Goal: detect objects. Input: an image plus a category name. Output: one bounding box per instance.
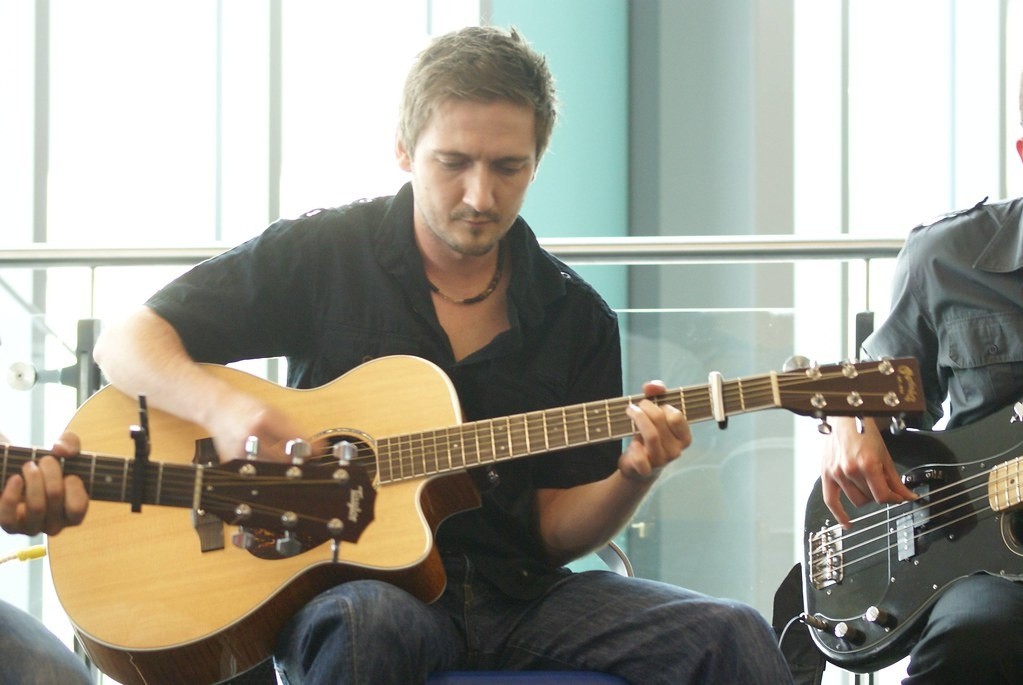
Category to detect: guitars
[802,398,1023,674]
[0,434,378,568]
[43,355,927,685]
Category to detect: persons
[0,430,96,685]
[92,26,793,685]
[821,73,1023,685]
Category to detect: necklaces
[427,239,505,305]
